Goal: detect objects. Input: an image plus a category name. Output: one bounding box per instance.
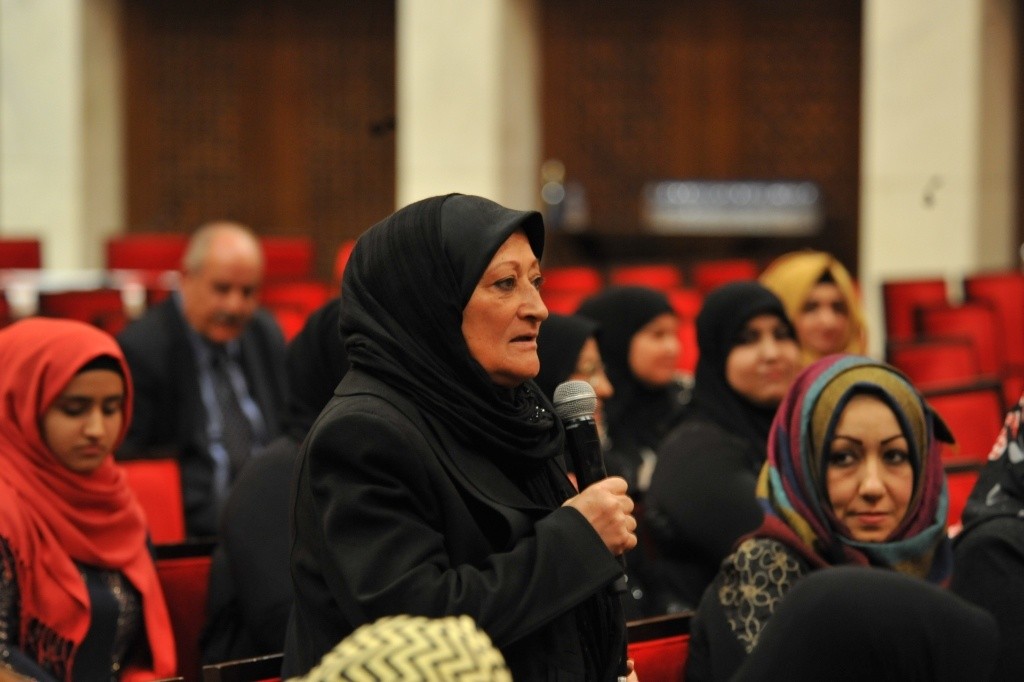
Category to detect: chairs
[1,227,1021,682]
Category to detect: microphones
[554,378,630,594]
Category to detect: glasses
[572,364,607,379]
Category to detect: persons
[682,351,1023,682]
[117,219,288,554]
[530,309,618,492]
[643,277,802,616]
[760,249,866,376]
[280,193,642,682]
[0,316,180,682]
[198,293,352,682]
[571,285,695,624]
